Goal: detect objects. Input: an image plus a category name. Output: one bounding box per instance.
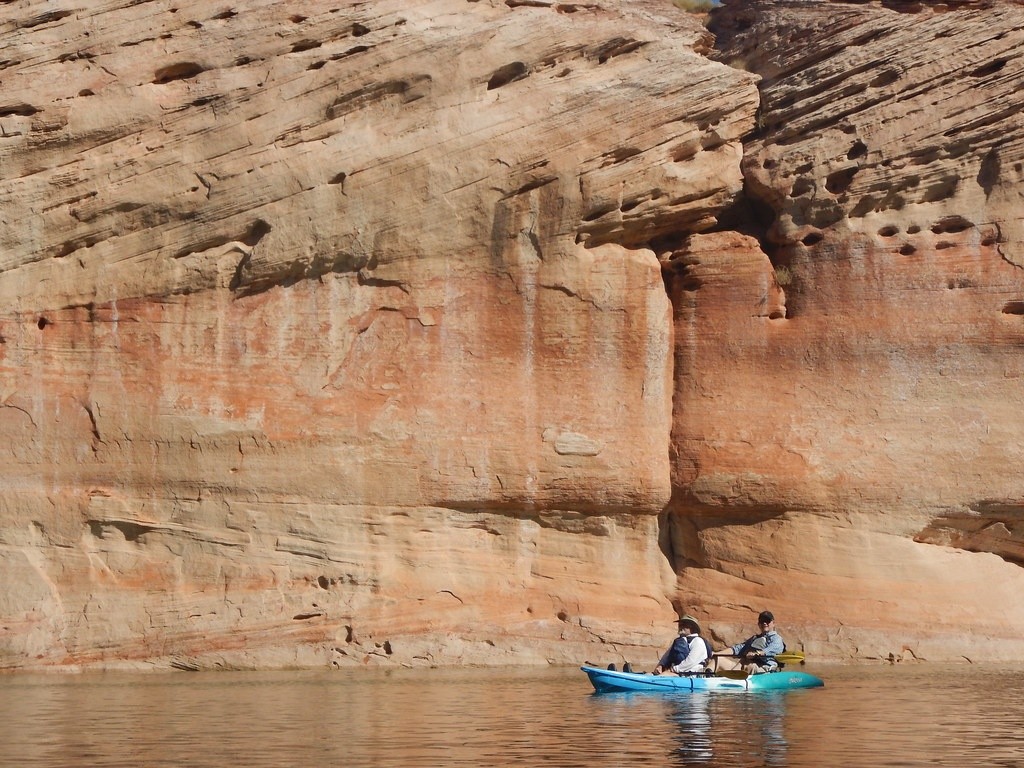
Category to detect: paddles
[714,649,806,664]
[635,669,749,681]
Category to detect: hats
[673,614,700,634]
[758,611,774,622]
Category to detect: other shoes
[705,668,715,678]
[623,663,632,673]
[607,663,617,671]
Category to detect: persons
[606,613,713,678]
[705,610,784,675]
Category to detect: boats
[580,665,825,691]
